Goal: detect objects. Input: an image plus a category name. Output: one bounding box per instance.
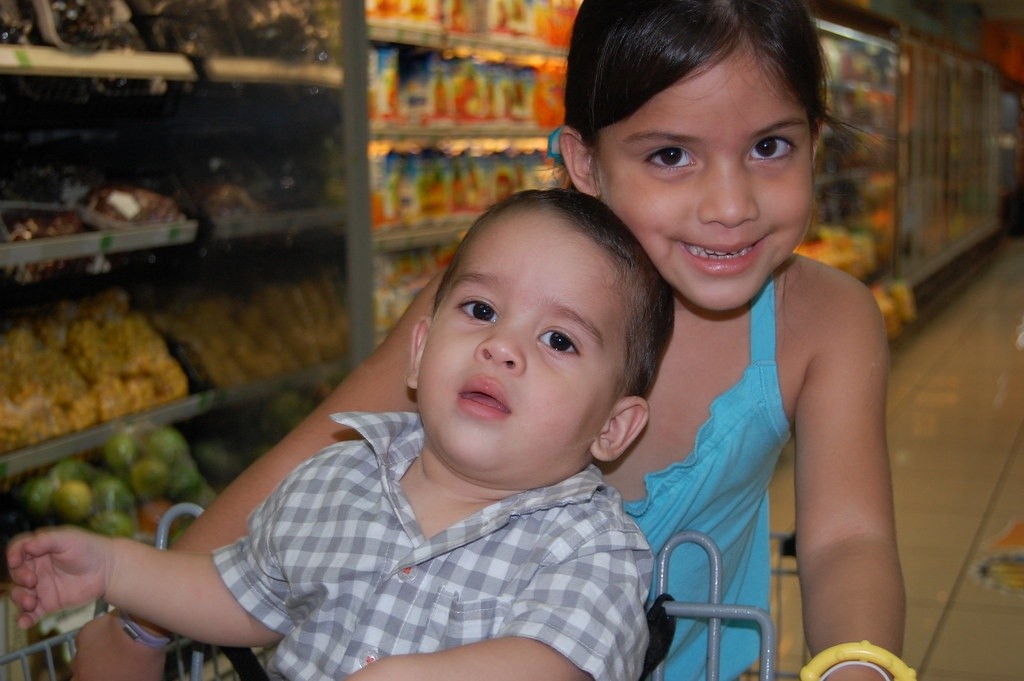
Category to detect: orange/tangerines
[18,381,337,550]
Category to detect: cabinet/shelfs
[0,0,376,681]
[359,0,904,349]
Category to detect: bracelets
[799,640,918,681]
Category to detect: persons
[7,187,676,681]
[69,0,906,681]
